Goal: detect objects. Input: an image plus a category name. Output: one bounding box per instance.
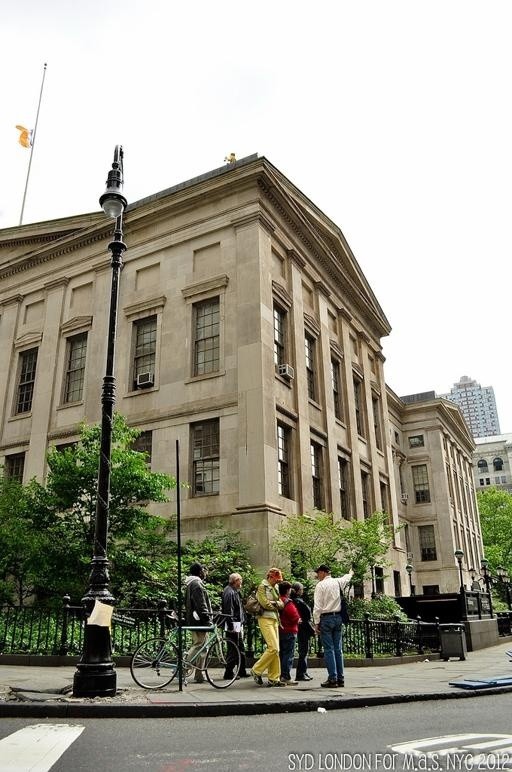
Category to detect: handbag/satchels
[342,596,349,626]
[245,594,264,615]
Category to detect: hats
[314,565,332,573]
[292,582,306,590]
[268,568,283,582]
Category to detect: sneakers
[223,675,240,680]
[237,673,251,678]
[194,678,206,683]
[171,668,184,681]
[251,671,312,686]
[337,678,344,686]
[321,680,338,688]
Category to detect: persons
[291,581,317,681]
[224,153,237,163]
[276,581,302,685]
[172,562,215,686]
[221,573,251,680]
[250,567,287,686]
[311,564,355,688]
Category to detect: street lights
[404,546,495,626]
[67,140,132,698]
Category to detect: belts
[321,612,340,615]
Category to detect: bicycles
[127,609,243,691]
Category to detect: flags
[15,125,34,149]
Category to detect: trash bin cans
[439,623,467,662]
